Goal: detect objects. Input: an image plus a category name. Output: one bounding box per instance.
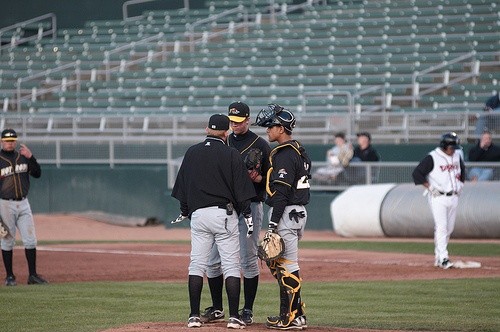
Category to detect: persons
[0,128,48,286]
[200,100,272,326]
[315,131,353,184]
[335,133,379,185]
[256,102,312,330]
[171,114,256,329]
[412,132,466,269]
[468,134,500,162]
[473,90,500,135]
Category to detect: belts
[439,191,457,196]
[218,206,227,209]
[2,196,26,201]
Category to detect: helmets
[440,132,463,150]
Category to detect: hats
[0,128,17,140]
[265,109,296,131]
[228,102,250,122]
[208,113,233,131]
[357,132,370,140]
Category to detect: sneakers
[188,311,204,327]
[203,307,225,323]
[239,309,254,325]
[435,258,454,270]
[272,316,308,330]
[27,274,48,284]
[6,274,16,286]
[227,315,246,328]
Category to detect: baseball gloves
[257,232,285,261]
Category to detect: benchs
[0,0,500,128]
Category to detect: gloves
[244,217,254,238]
[429,186,438,198]
[264,221,278,242]
[171,212,189,224]
